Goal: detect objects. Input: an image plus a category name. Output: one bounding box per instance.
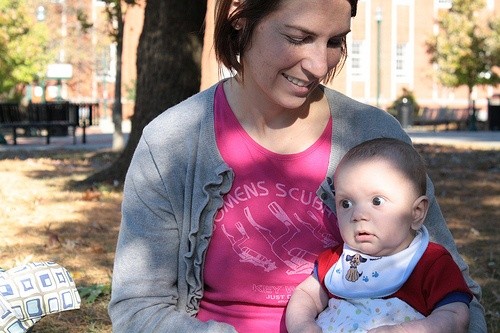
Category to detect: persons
[284,137,473,333]
[107,0,487,333]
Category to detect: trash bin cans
[398,97,413,128]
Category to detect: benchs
[415,107,480,133]
[0,101,99,146]
